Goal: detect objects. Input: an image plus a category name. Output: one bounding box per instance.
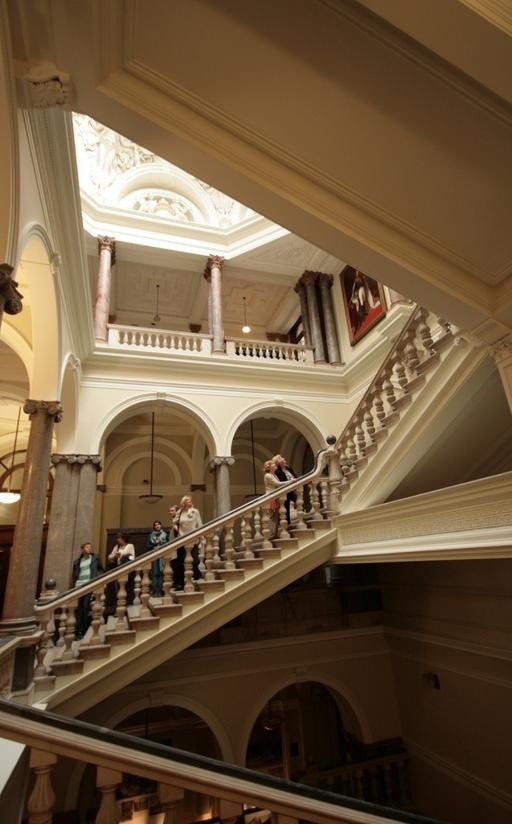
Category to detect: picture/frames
[338,263,390,346]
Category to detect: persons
[262,460,296,540]
[351,271,371,322]
[107,531,136,618]
[72,543,104,641]
[272,454,298,530]
[145,521,169,595]
[169,505,180,541]
[172,495,203,591]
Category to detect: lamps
[135,407,263,508]
[0,402,22,508]
